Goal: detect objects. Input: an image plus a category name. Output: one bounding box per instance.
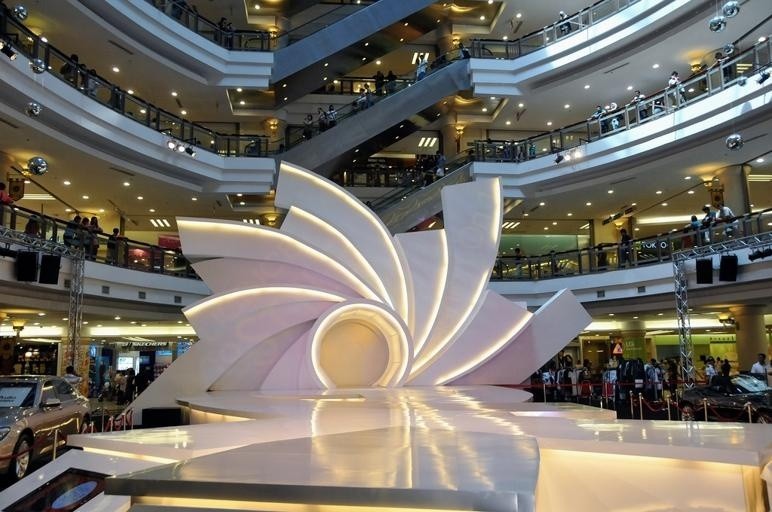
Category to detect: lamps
[167,140,196,157]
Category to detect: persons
[759,459,772,512]
[23,215,40,237]
[112,368,149,402]
[63,216,102,262]
[643,357,695,403]
[619,229,633,268]
[62,366,83,389]
[682,202,738,248]
[539,358,595,405]
[591,103,622,133]
[494,252,502,279]
[2,182,13,204]
[751,354,769,374]
[108,228,120,265]
[514,248,524,277]
[597,246,607,271]
[153,2,234,50]
[707,53,734,83]
[670,71,687,107]
[558,10,572,35]
[630,90,649,120]
[58,53,98,97]
[278,41,493,211]
[703,357,731,386]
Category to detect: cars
[1,374,93,480]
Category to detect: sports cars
[680,375,772,423]
